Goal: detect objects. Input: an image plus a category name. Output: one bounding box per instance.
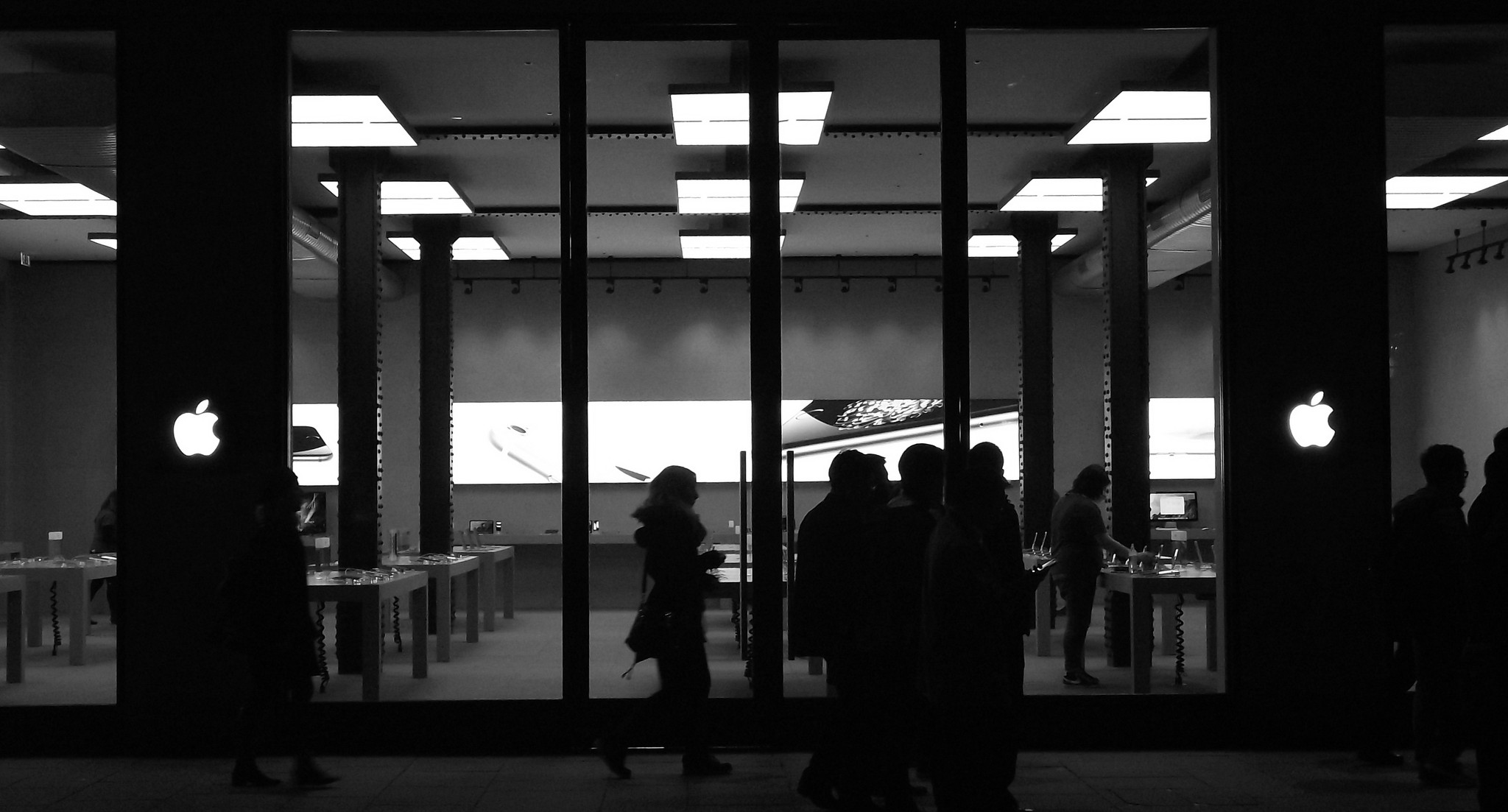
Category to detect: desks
[1099,562,1218,694]
[705,541,753,653]
[304,563,432,700]
[450,544,517,631]
[0,551,119,686]
[377,553,481,663]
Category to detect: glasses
[1461,470,1469,478]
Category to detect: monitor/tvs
[1149,491,1198,521]
[298,492,327,534]
[469,520,494,534]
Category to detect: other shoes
[289,756,342,790]
[1418,759,1475,791]
[231,754,281,787]
[796,749,1018,812]
[90,619,98,625]
[1356,745,1405,766]
[1062,670,1100,689]
[681,751,733,777]
[595,732,633,778]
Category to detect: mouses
[1201,528,1209,530]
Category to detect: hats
[262,467,298,496]
[897,442,945,481]
[969,442,1005,477]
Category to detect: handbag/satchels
[625,600,682,662]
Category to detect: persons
[1051,463,1154,689]
[595,465,733,776]
[1464,427,1508,810]
[229,467,339,791]
[790,442,1051,812]
[1392,444,1473,788]
[87,489,116,626]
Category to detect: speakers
[593,520,600,530]
[496,521,502,531]
